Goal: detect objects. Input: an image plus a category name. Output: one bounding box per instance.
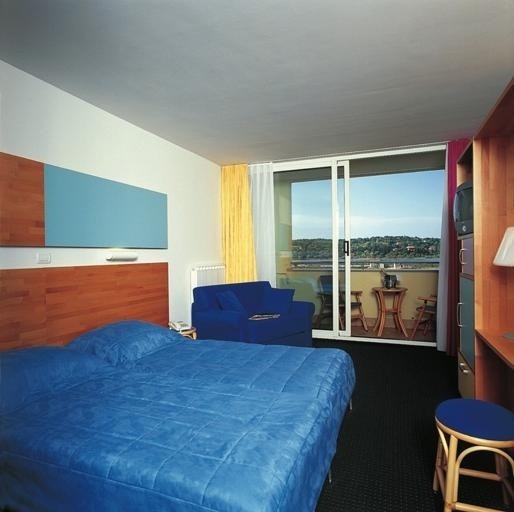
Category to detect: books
[249,314,280,321]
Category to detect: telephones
[167,320,190,331]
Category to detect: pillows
[0,316,183,416]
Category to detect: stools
[316,275,438,340]
[433,398,514,512]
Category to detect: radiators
[192,264,226,288]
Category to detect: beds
[1,261,357,512]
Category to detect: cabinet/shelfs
[456,75,514,409]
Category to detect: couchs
[192,280,315,348]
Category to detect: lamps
[107,252,138,262]
[492,227,514,268]
